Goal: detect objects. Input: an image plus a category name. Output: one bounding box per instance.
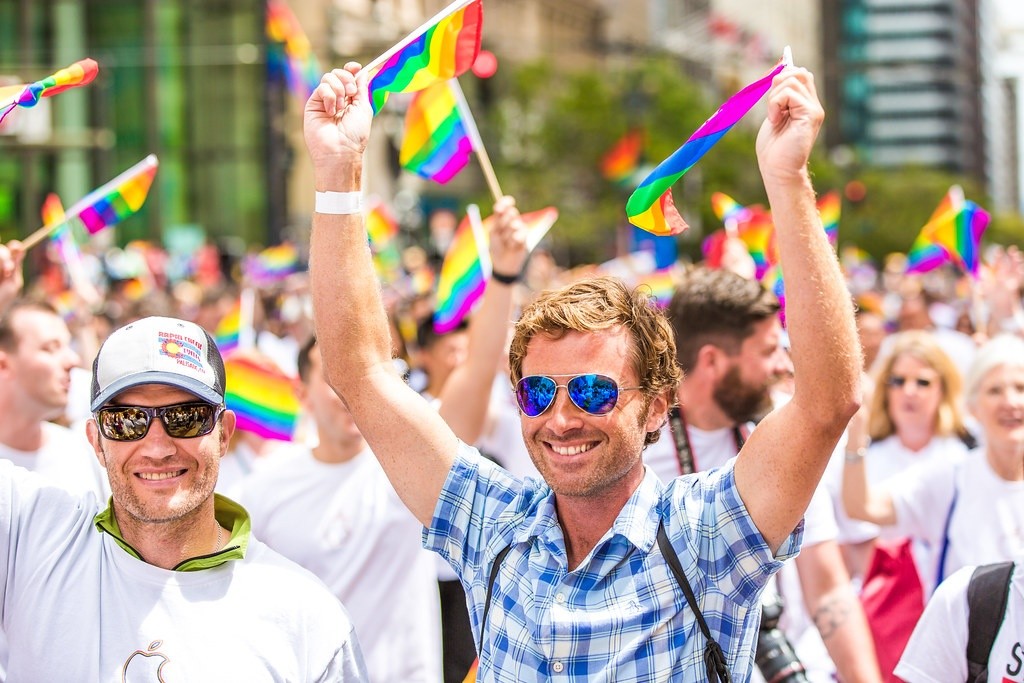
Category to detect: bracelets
[490,271,520,286]
[844,450,864,464]
[311,191,364,215]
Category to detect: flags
[218,348,301,442]
[904,185,991,279]
[361,0,484,185]
[624,62,787,239]
[43,153,157,240]
[0,57,98,114]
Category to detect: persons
[102,410,146,439]
[163,406,213,427]
[0,200,1024,683]
[301,60,865,683]
[523,380,555,410]
[0,238,367,683]
[571,385,618,408]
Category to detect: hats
[90,316,226,412]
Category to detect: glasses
[94,403,226,442]
[512,373,646,417]
